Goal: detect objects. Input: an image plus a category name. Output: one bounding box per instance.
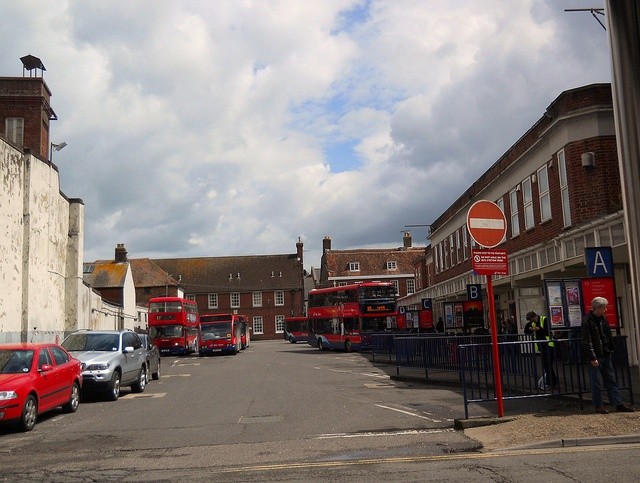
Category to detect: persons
[524,311,556,390]
[581,296,636,413]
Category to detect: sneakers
[595,405,608,414]
[616,403,635,412]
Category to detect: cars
[137,334,161,384]
[0,343,83,431]
[59,328,147,401]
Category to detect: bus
[198,314,241,357]
[308,281,398,353]
[237,315,250,350]
[284,317,308,343]
[147,297,199,356]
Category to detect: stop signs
[467,200,508,249]
